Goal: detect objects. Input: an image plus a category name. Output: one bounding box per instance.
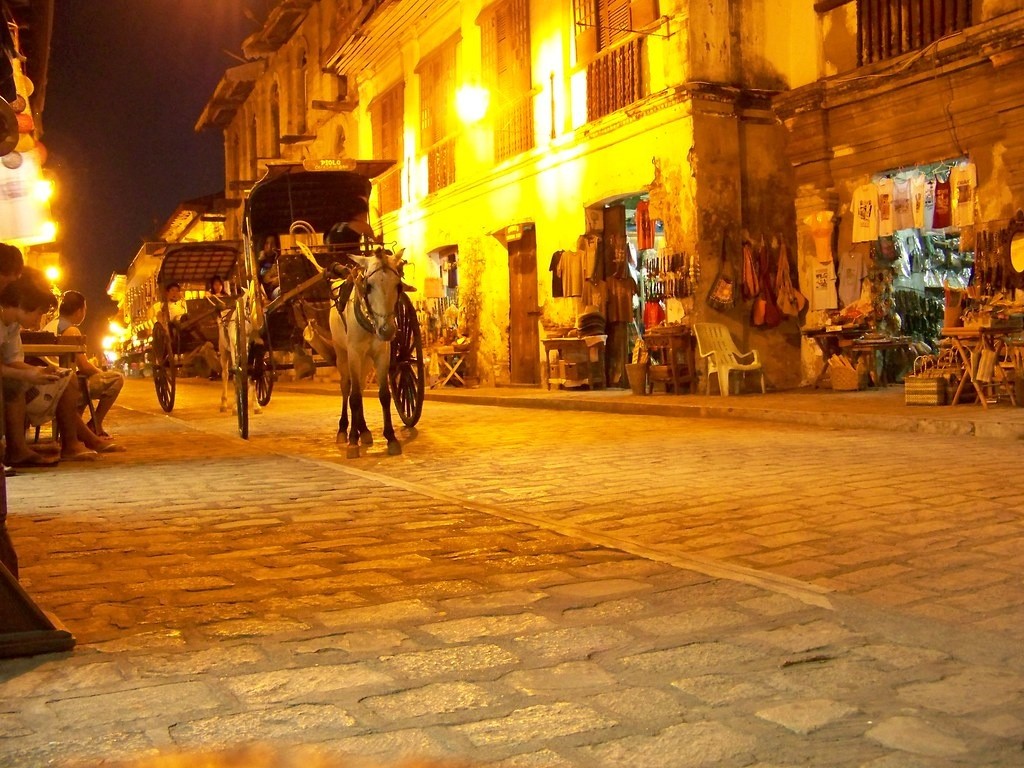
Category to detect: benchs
[186,299,220,342]
[280,251,340,301]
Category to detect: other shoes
[4,466,16,476]
[4,452,59,467]
[98,431,115,440]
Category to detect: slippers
[58,450,104,462]
[98,444,126,453]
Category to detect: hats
[776,285,807,313]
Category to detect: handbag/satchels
[706,274,736,312]
[743,243,760,296]
[752,297,767,326]
[764,303,784,328]
[740,279,751,302]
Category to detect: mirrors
[998,212,1024,289]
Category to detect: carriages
[104,237,278,418]
[228,156,429,459]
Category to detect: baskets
[904,344,972,405]
[827,362,867,392]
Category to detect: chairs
[35,374,105,443]
[694,322,765,398]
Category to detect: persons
[0,241,145,475]
[167,285,213,348]
[203,275,230,297]
[803,211,838,269]
[257,233,282,275]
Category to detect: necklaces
[2,304,12,343]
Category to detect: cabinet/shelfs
[541,335,607,392]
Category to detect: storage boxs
[649,364,671,379]
[963,316,1023,328]
[558,360,578,380]
[550,364,559,378]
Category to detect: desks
[806,332,875,384]
[940,328,1020,406]
[438,350,472,387]
[643,333,697,396]
[25,343,88,443]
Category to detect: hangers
[863,173,869,184]
[932,159,954,174]
[914,162,921,172]
[881,167,888,179]
[898,164,904,173]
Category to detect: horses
[329,248,416,459]
[217,279,262,415]
